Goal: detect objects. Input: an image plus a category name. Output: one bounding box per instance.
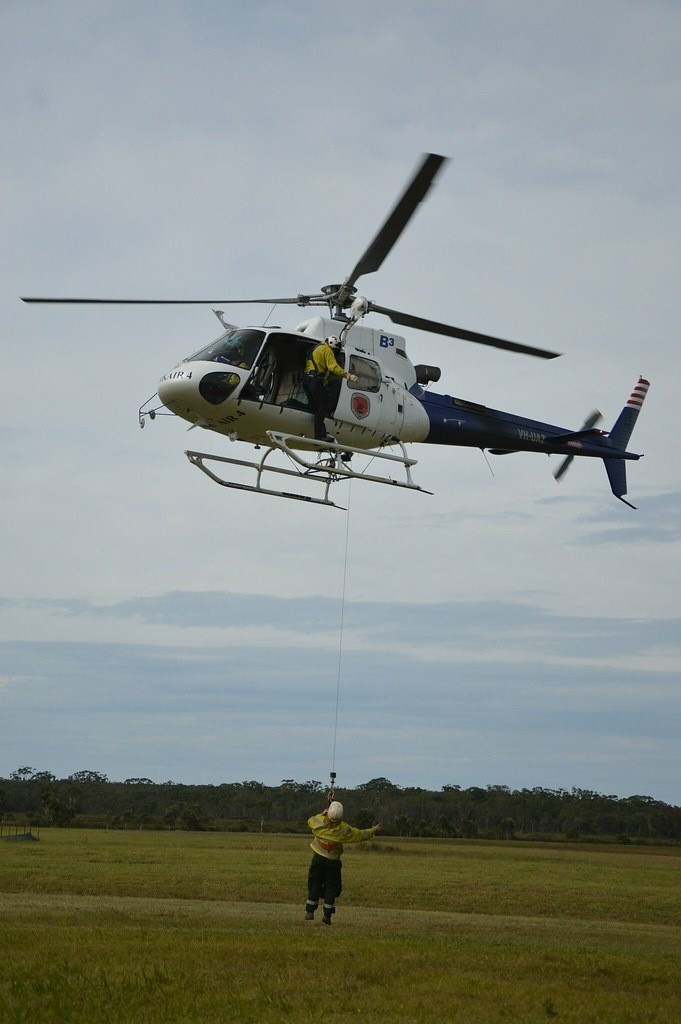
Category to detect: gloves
[347,373,359,384]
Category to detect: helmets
[328,335,338,348]
[328,801,343,822]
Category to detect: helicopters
[22,153,651,512]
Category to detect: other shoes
[313,436,334,446]
[322,916,331,925]
[305,913,314,920]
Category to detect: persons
[218,352,251,396]
[304,802,382,924]
[303,335,359,445]
